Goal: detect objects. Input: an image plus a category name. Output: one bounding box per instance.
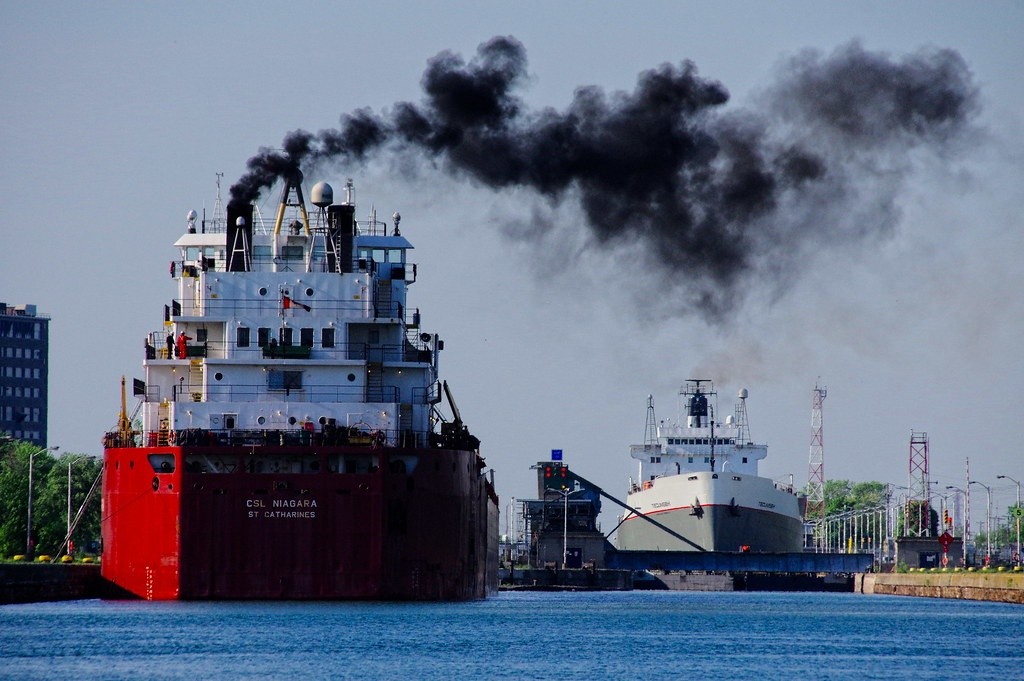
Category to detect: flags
[283,296,312,312]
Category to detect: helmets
[171,332,174,335]
[181,331,185,334]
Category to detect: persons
[166,331,192,360]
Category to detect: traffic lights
[67,540,74,555]
[545,466,569,482]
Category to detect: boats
[101,159,501,601]
[618,379,803,553]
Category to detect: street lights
[66,455,97,554]
[547,487,586,563]
[805,475,1020,568]
[26,446,60,561]
